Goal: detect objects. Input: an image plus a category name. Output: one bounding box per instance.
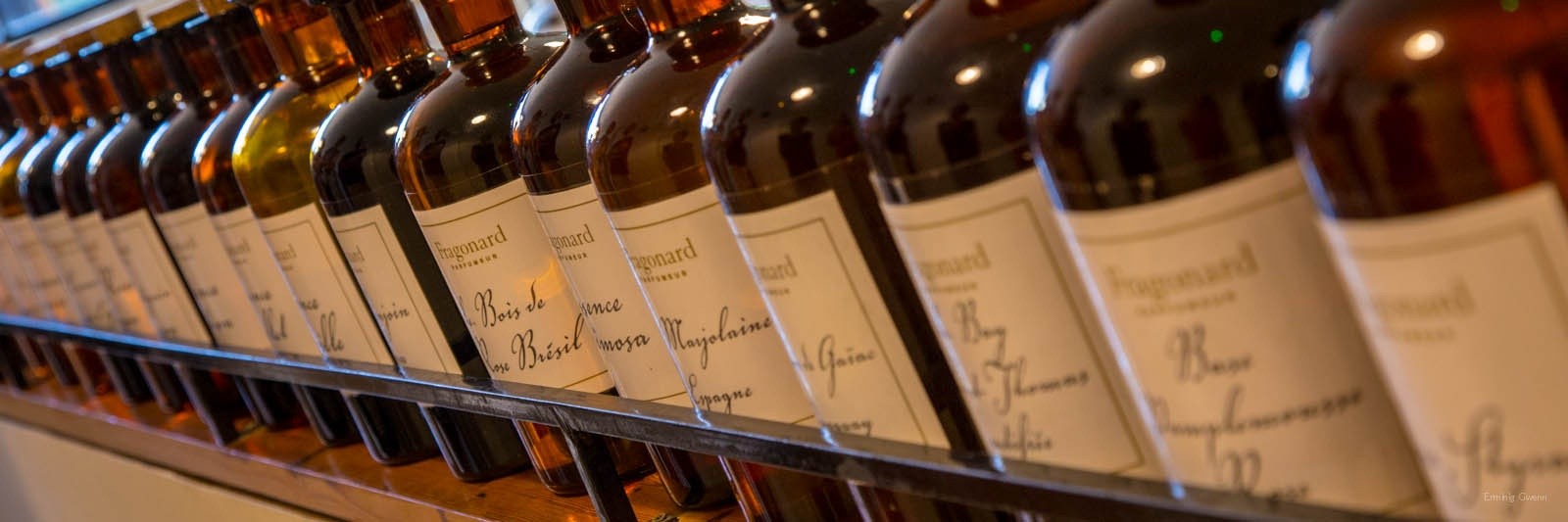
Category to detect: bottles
[1020,0,1440,522]
[699,0,1010,522]
[0,0,189,418]
[508,0,738,512]
[135,0,306,434]
[854,0,1166,522]
[308,0,530,485]
[230,0,443,466]
[189,0,361,449]
[1279,0,1568,522]
[392,0,658,500]
[584,0,853,522]
